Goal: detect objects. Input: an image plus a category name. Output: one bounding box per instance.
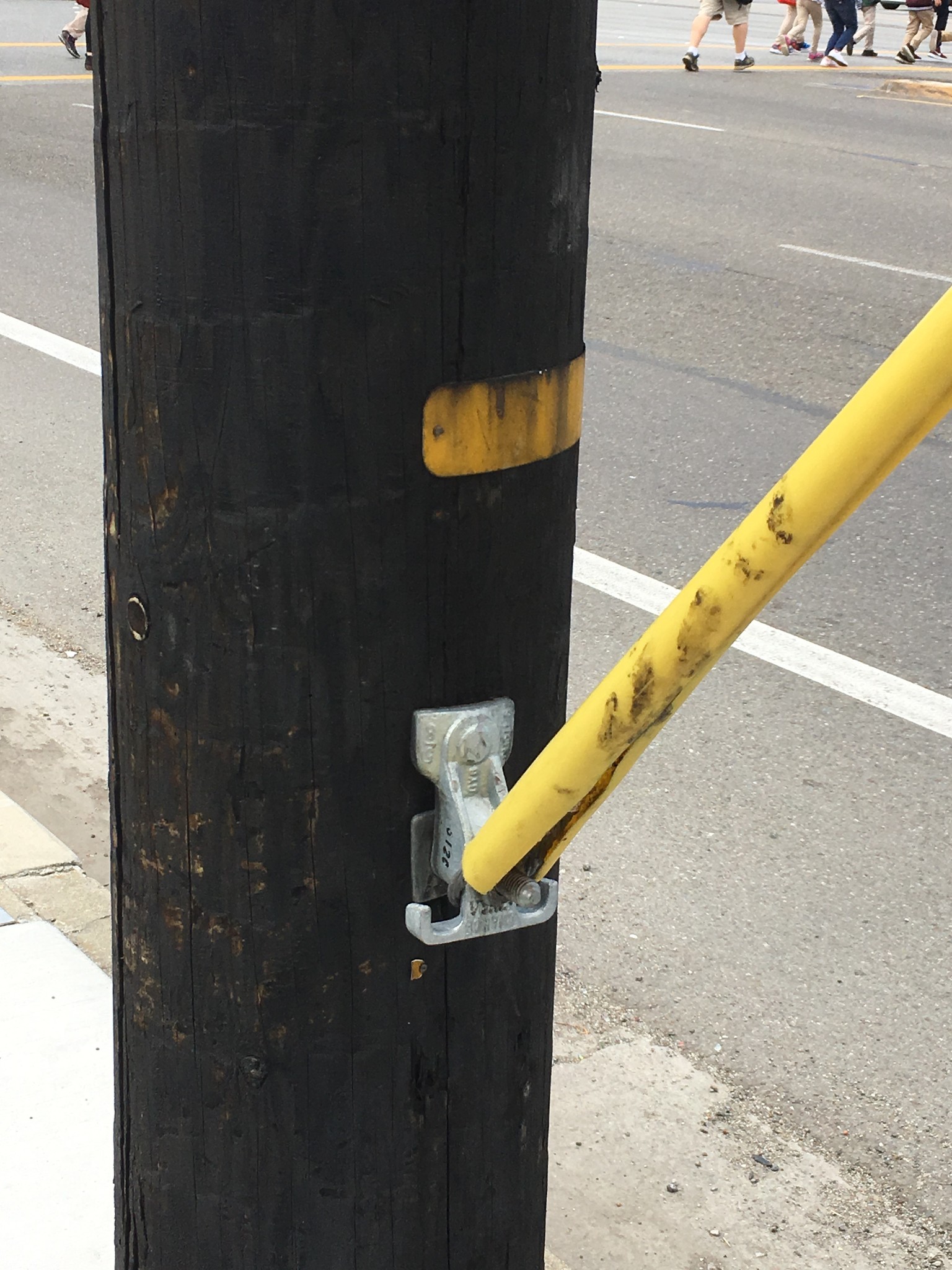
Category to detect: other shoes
[846,37,856,55]
[929,30,942,51]
[862,50,877,56]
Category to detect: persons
[682,0,755,71]
[58,0,93,70]
[769,0,952,69]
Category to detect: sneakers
[807,52,823,61]
[828,49,847,66]
[85,54,93,69]
[771,44,783,54]
[682,53,700,71]
[928,50,947,60]
[820,56,838,67]
[913,54,922,60]
[895,52,912,64]
[59,31,80,58]
[734,56,754,70]
[800,42,810,50]
[903,43,915,62]
[789,40,802,53]
[780,35,790,56]
